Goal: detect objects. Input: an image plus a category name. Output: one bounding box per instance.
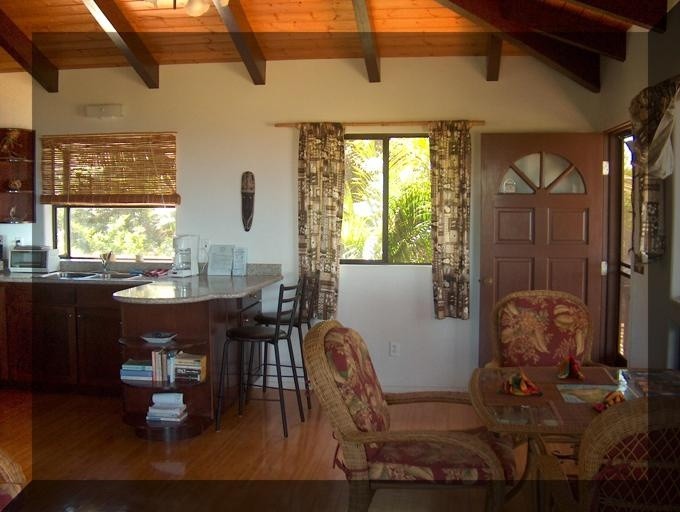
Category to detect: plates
[141,330,178,344]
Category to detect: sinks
[86,273,143,281]
[42,271,97,281]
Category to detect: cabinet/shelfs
[238,289,262,392]
[119,336,208,430]
[6,283,139,395]
[0,128,35,224]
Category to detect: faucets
[100,250,113,274]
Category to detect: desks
[468,366,680,512]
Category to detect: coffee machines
[168,233,201,278]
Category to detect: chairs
[245,269,320,410]
[0,447,32,512]
[531,397,680,512]
[484,290,618,367]
[215,278,305,437]
[302,321,516,512]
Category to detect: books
[620,366,680,403]
[119,348,207,421]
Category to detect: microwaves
[7,248,61,274]
